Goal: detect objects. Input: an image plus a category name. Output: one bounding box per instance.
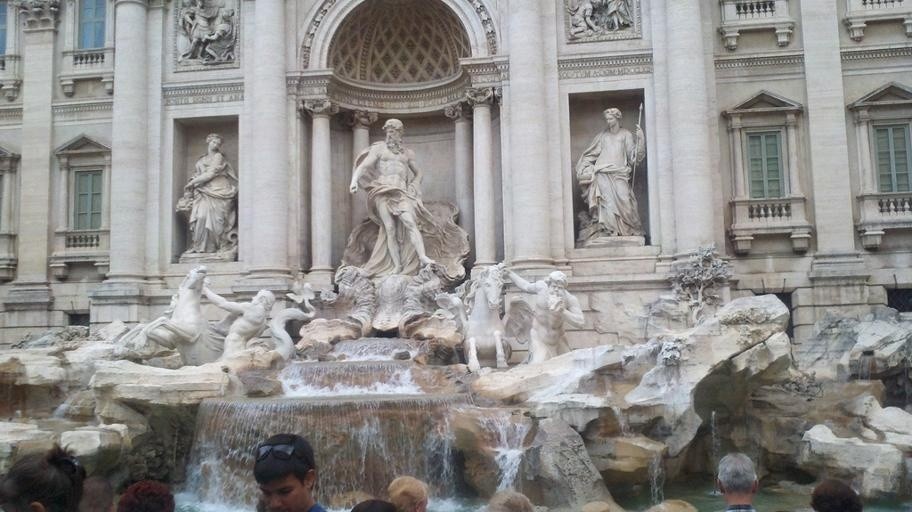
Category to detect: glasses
[257,444,295,460]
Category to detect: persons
[78,477,114,512]
[810,478,863,512]
[351,117,468,275]
[179,0,236,63]
[183,133,238,252]
[388,476,428,512]
[118,482,175,512]
[0,445,86,512]
[575,108,647,238]
[566,0,635,42]
[202,280,315,367]
[490,491,534,512]
[502,266,585,365]
[718,452,760,512]
[350,499,395,512]
[255,433,326,512]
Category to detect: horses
[117,264,225,365]
[460,261,511,374]
